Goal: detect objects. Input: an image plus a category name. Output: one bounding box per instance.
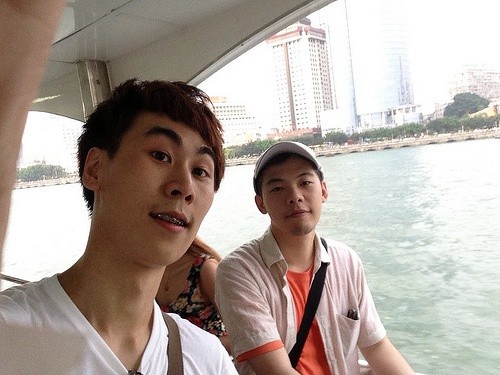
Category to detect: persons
[215,142,415,375]
[0,0,239,375]
[155,235,232,356]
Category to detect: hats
[253,142,325,182]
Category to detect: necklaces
[165,267,171,291]
